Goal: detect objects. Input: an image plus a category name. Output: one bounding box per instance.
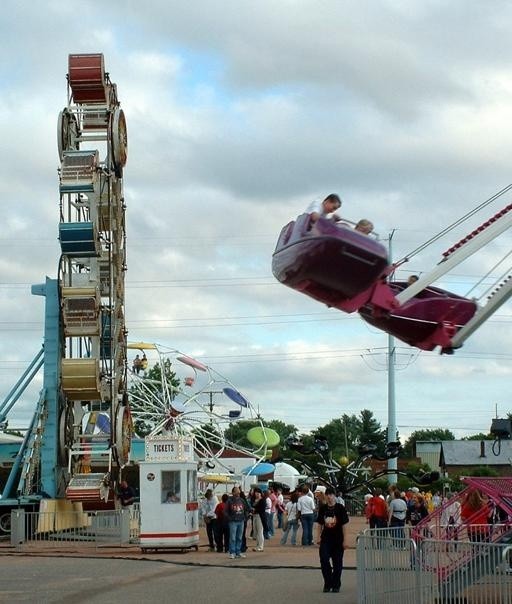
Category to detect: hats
[408,487,419,493]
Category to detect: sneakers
[209,547,263,559]
[332,588,339,592]
[323,587,331,591]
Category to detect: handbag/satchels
[283,516,287,532]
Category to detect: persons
[364,486,462,553]
[356,220,374,235]
[165,492,175,504]
[405,275,419,286]
[304,194,342,222]
[461,488,509,559]
[201,488,266,559]
[132,354,147,375]
[315,488,349,593]
[263,485,345,546]
[116,479,135,521]
